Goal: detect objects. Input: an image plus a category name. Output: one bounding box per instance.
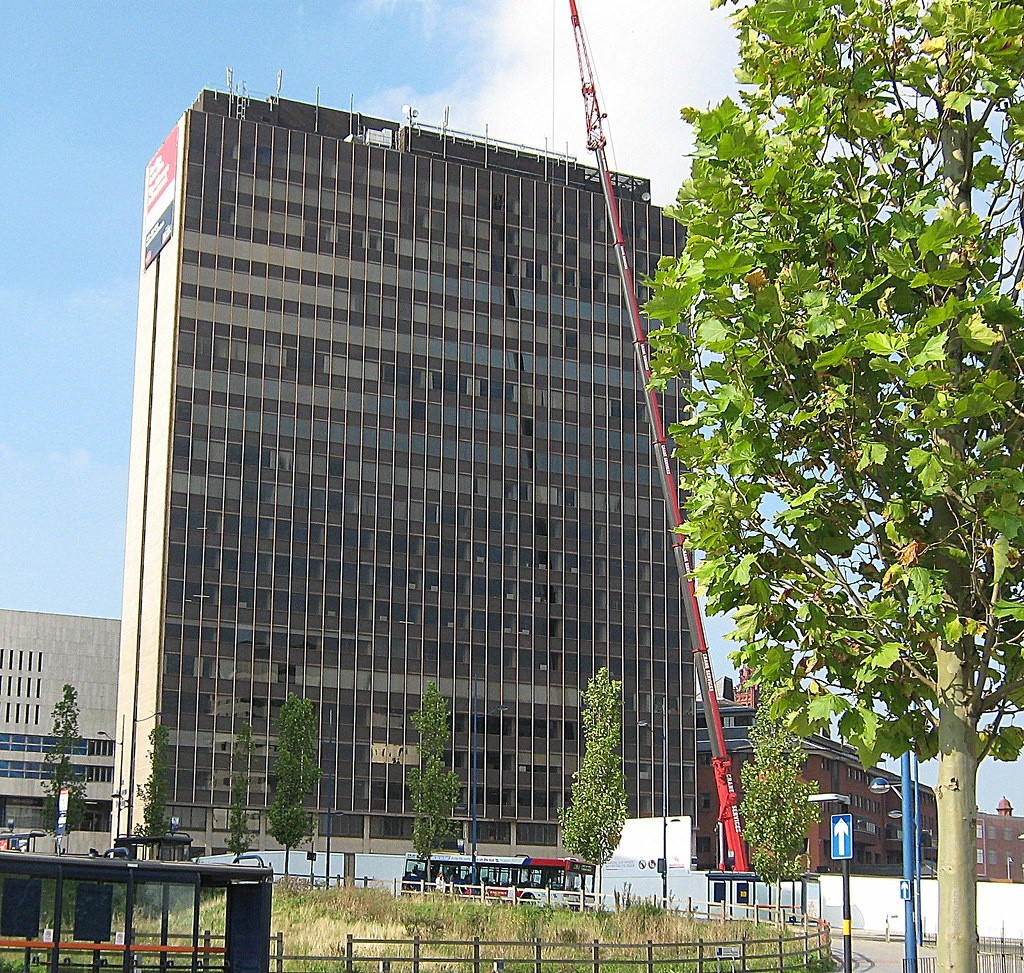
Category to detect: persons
[435,872,446,894]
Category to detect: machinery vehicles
[569,1,750,872]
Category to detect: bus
[400,852,595,913]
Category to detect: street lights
[326,811,344,887]
[638,697,668,908]
[472,673,508,894]
[128,711,169,837]
[869,776,923,948]
[97,714,126,838]
